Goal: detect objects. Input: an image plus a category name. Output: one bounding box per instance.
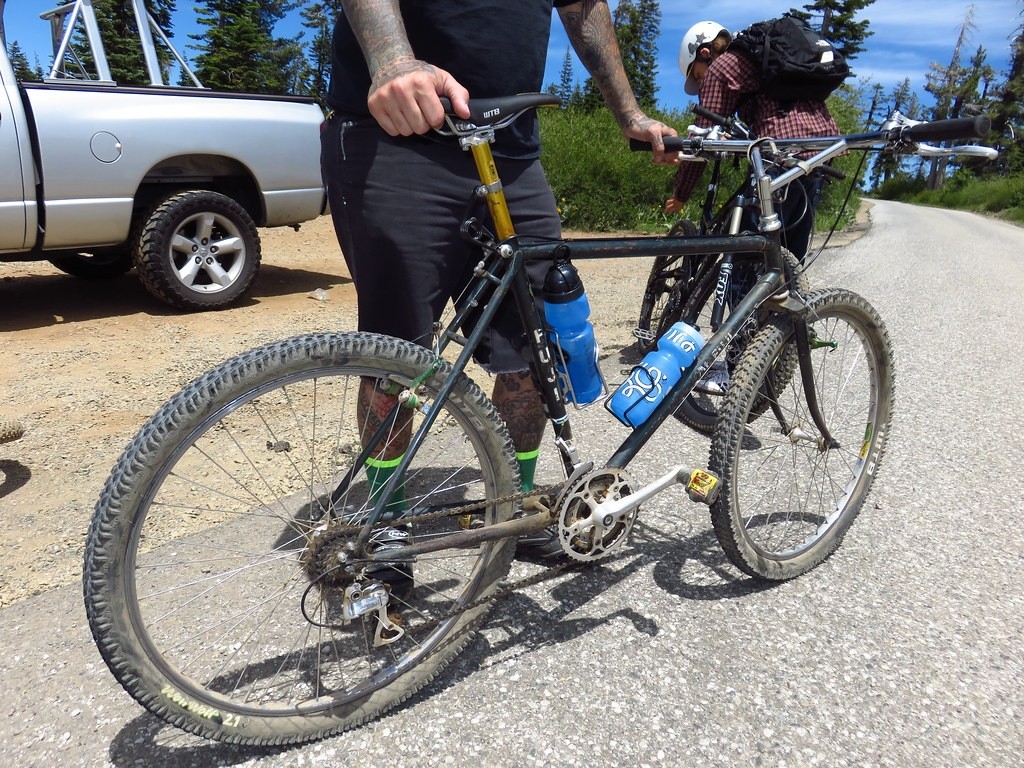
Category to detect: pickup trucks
[0,1,333,310]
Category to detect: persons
[661,16,850,391]
[321,0,681,616]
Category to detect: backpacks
[727,11,850,116]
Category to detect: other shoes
[513,511,577,560]
[370,523,416,603]
[693,367,729,395]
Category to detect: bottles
[541,244,603,405]
[609,321,704,425]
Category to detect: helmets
[678,20,732,95]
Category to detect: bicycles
[82,92,895,748]
[631,103,848,432]
